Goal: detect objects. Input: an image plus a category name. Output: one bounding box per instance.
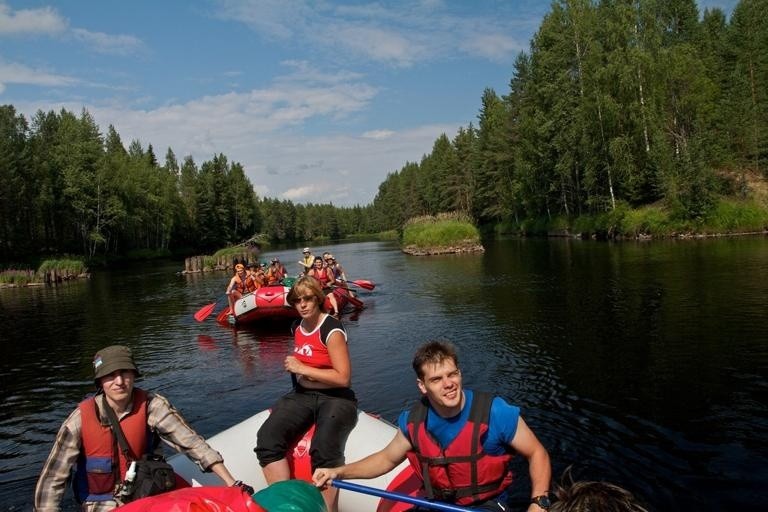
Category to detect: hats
[303,248,311,254]
[324,255,335,260]
[235,256,279,269]
[92,345,136,380]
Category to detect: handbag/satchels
[121,453,176,504]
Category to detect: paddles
[217,280,255,325]
[322,281,364,309]
[194,274,250,322]
[339,279,375,290]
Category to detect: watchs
[531,495,551,508]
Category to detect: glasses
[292,295,316,304]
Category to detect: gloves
[232,481,254,495]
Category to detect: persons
[547,464,645,510]
[309,338,552,512]
[224,247,356,319]
[30,343,254,511]
[254,275,360,511]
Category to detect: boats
[161,402,427,512]
[230,284,349,327]
